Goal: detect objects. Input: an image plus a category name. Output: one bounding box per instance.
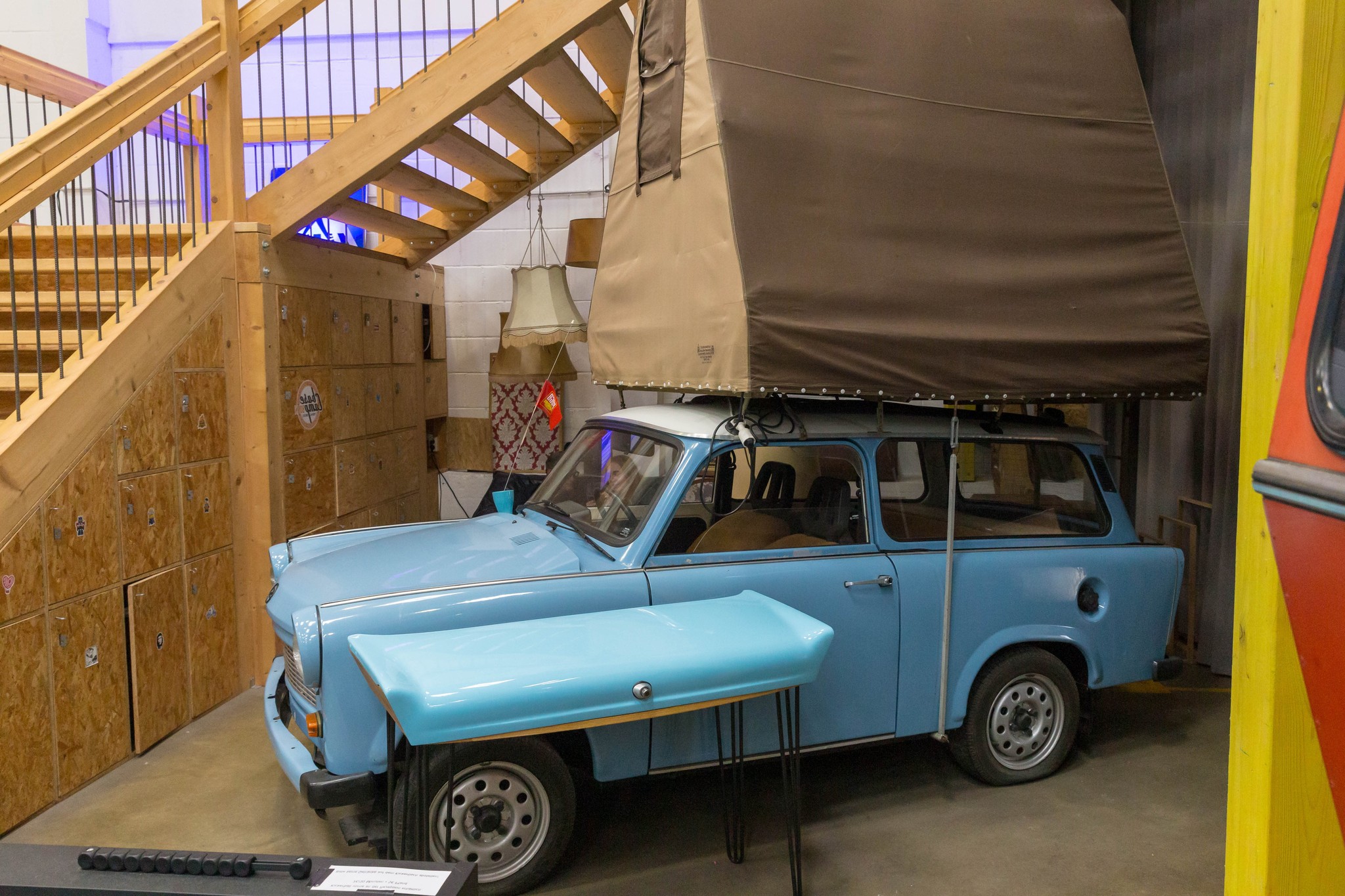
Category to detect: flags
[535,379,563,431]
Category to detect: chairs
[682,461,850,554]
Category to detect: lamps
[487,192,578,382]
[564,101,606,268]
[502,184,587,350]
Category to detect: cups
[492,489,514,514]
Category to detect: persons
[585,454,647,508]
[512,451,583,515]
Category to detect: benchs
[880,499,1076,536]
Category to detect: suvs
[258,396,1184,896]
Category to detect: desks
[347,591,835,896]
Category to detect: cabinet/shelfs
[0,282,492,840]
